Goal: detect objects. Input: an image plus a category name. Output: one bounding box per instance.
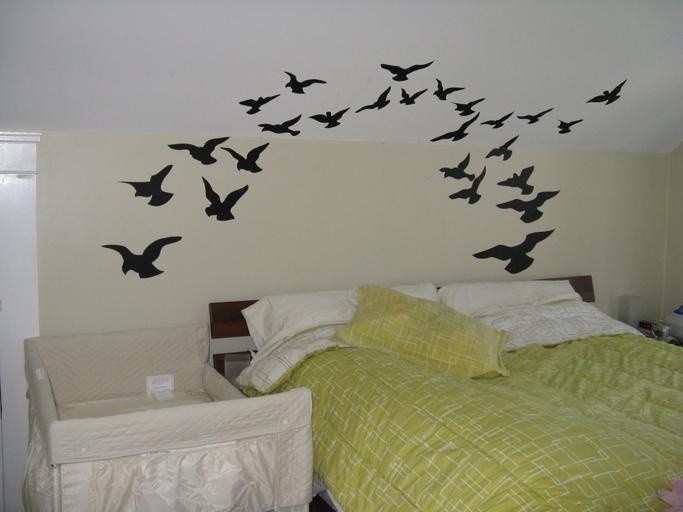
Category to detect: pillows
[336,284,510,378]
[242,283,440,356]
[436,280,585,318]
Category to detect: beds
[208,275,683,512]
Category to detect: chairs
[18,321,313,512]
